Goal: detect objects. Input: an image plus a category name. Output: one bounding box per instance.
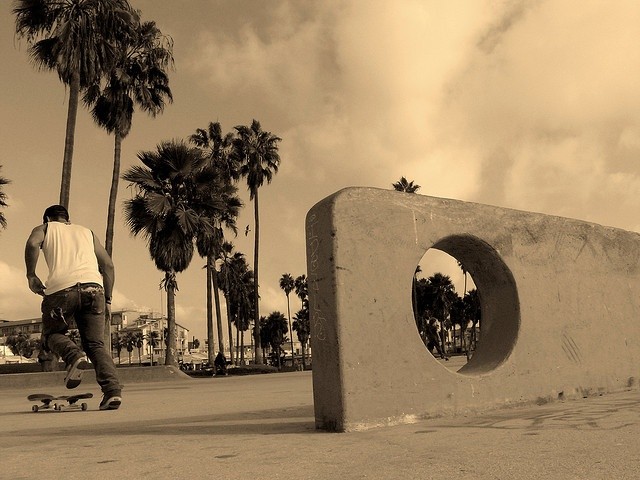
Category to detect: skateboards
[28,393,93,411]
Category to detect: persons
[24,204,122,410]
[212,352,228,375]
[424,317,449,359]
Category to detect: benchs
[201,361,240,376]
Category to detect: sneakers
[64,355,88,389]
[99,389,122,411]
